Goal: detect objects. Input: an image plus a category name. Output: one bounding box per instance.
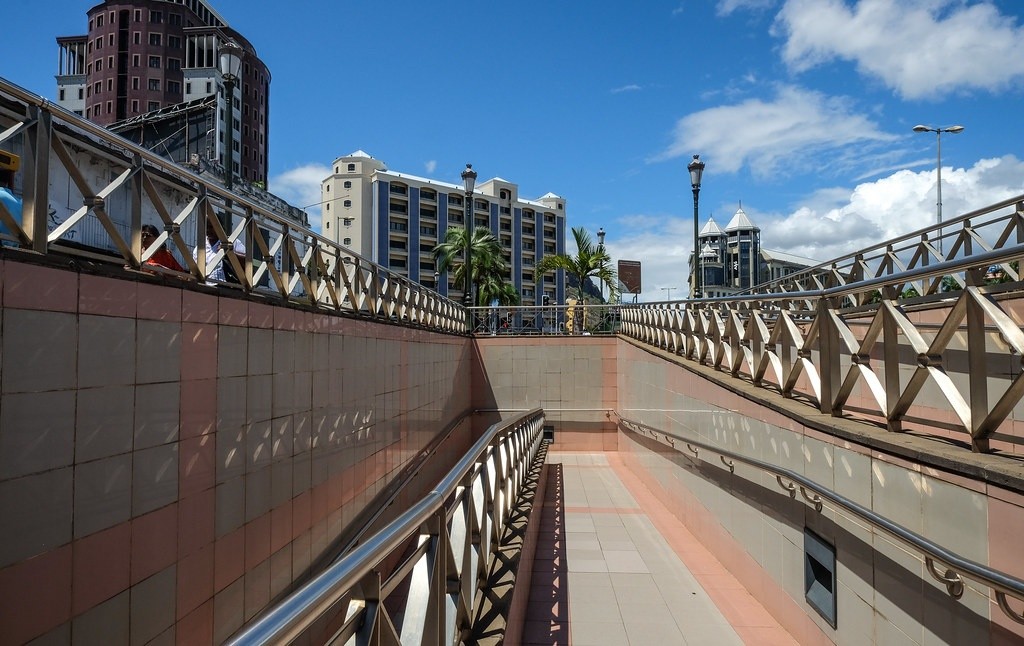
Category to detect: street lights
[687,154,706,298]
[336,216,355,243]
[460,163,478,333]
[218,36,244,235]
[597,227,606,305]
[912,124,964,293]
[435,271,439,293]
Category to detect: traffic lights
[542,295,550,313]
[507,312,512,324]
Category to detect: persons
[141,224,184,275]
[583,329,591,336]
[503,321,508,335]
[191,213,246,288]
